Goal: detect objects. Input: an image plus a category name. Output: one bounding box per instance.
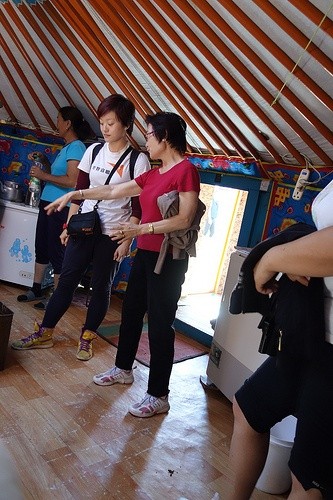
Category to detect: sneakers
[10,321,53,350]
[92,367,134,387]
[75,330,98,360]
[127,393,170,418]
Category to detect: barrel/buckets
[254,415,297,494]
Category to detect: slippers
[33,301,48,309]
[17,291,45,302]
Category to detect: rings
[32,171,34,174]
[122,235,125,238]
[120,231,123,235]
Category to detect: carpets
[81,319,210,370]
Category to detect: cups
[25,188,40,206]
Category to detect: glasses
[143,130,158,140]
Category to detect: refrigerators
[206,246,270,406]
[0,199,40,288]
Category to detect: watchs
[63,223,68,230]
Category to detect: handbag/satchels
[65,211,102,237]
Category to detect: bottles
[28,154,43,194]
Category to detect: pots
[0,180,26,202]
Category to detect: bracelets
[148,222,155,235]
[78,190,84,201]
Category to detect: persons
[16,105,91,311]
[11,92,152,361]
[44,110,201,419]
[211,174,333,500]
